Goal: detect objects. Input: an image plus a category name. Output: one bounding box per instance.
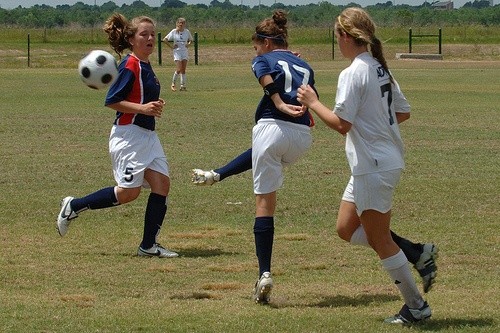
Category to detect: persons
[163,17,193,90]
[190,11,319,305]
[296,8,441,327]
[55,13,180,259]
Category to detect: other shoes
[171,84,177,91]
[180,85,187,91]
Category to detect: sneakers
[252,272,273,304]
[412,243,439,293]
[137,243,179,258]
[188,168,221,188]
[54,195,79,237]
[383,300,432,326]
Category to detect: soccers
[78,50,119,91]
